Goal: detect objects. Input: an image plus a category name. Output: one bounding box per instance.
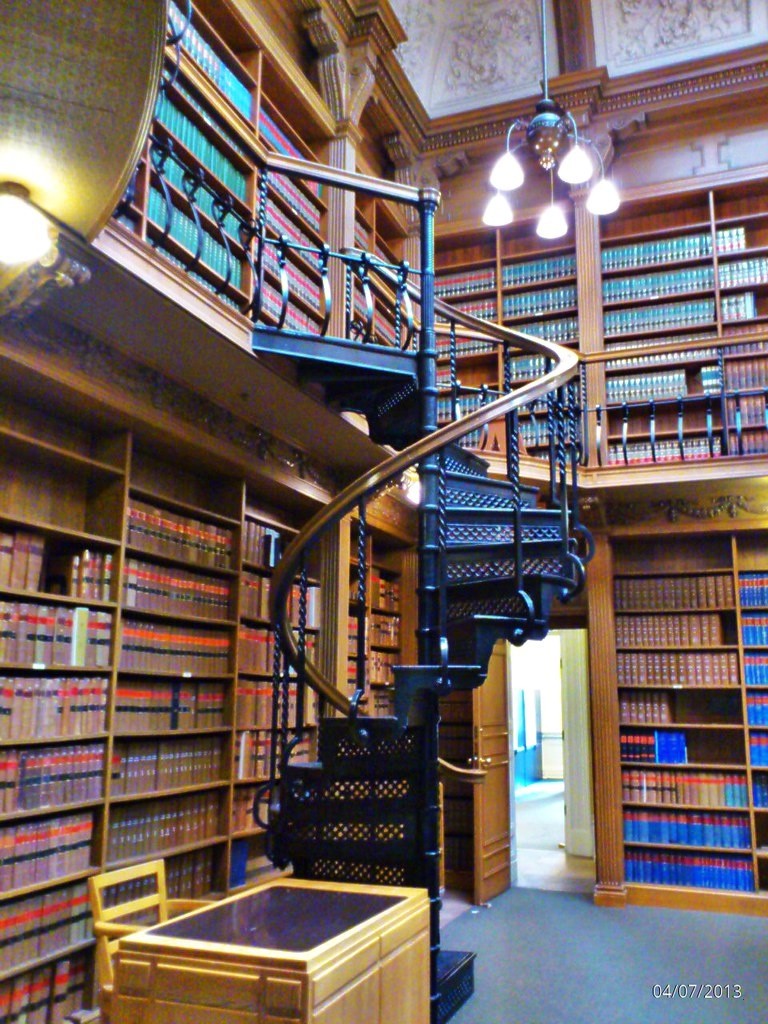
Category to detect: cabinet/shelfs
[579,495,768,915]
[0,349,474,1024]
[99,0,768,475]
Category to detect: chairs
[86,858,217,1024]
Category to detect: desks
[109,878,432,1024]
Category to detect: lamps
[483,0,621,239]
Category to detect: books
[0,496,235,1024]
[607,395,768,465]
[613,574,768,892]
[228,522,401,889]
[601,226,768,404]
[259,104,322,333]
[434,255,579,462]
[355,219,406,345]
[122,1,254,312]
